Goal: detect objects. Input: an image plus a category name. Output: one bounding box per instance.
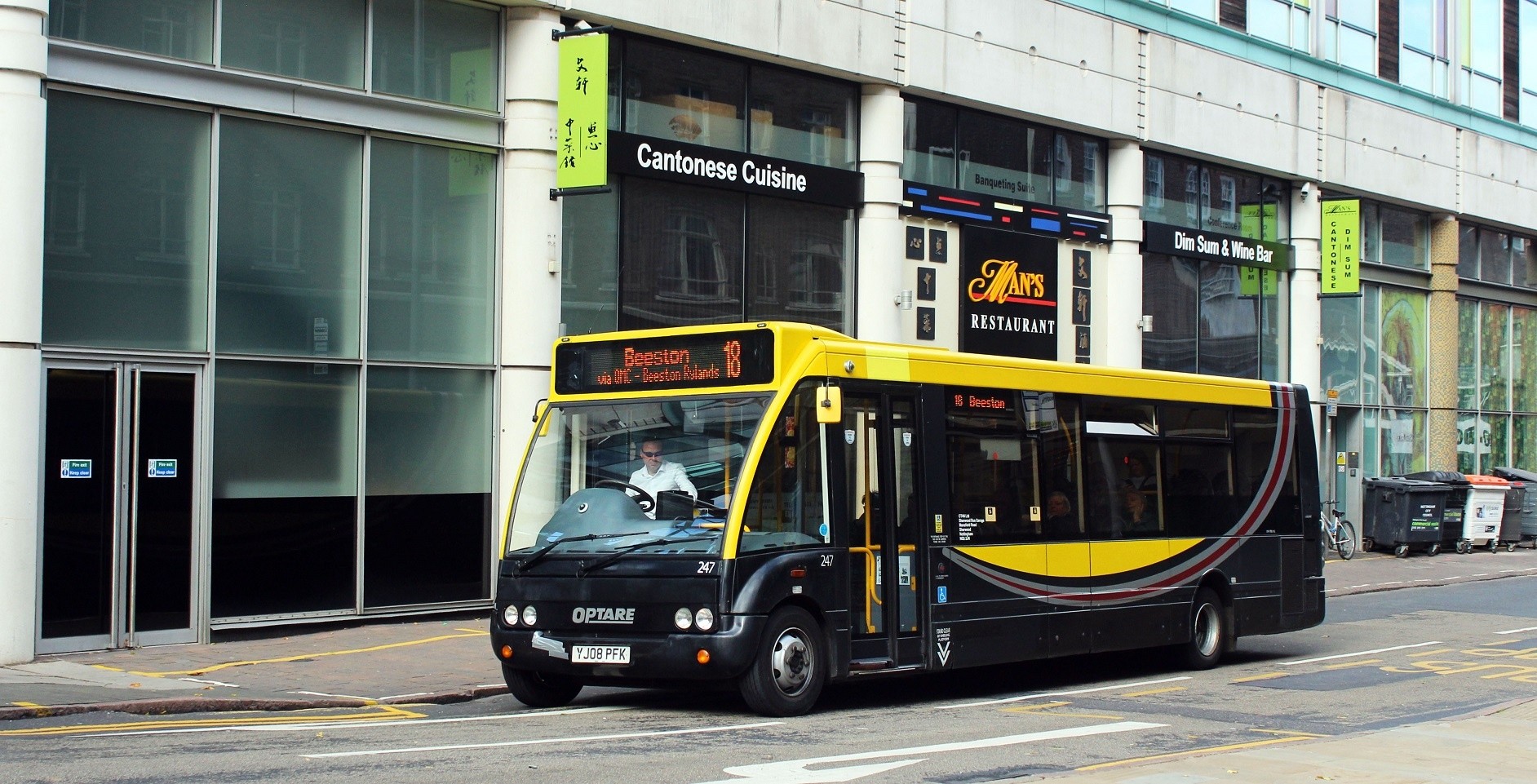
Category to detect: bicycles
[1319,500,1356,561]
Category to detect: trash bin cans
[1489,483,1529,552]
[1490,467,1537,549]
[1461,475,1511,553]
[1394,471,1475,554]
[1361,477,1453,558]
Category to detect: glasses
[642,448,663,457]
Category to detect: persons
[855,488,918,545]
[1112,489,1160,540]
[624,439,698,519]
[1034,488,1079,544]
[953,449,1157,535]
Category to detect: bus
[489,320,1327,716]
